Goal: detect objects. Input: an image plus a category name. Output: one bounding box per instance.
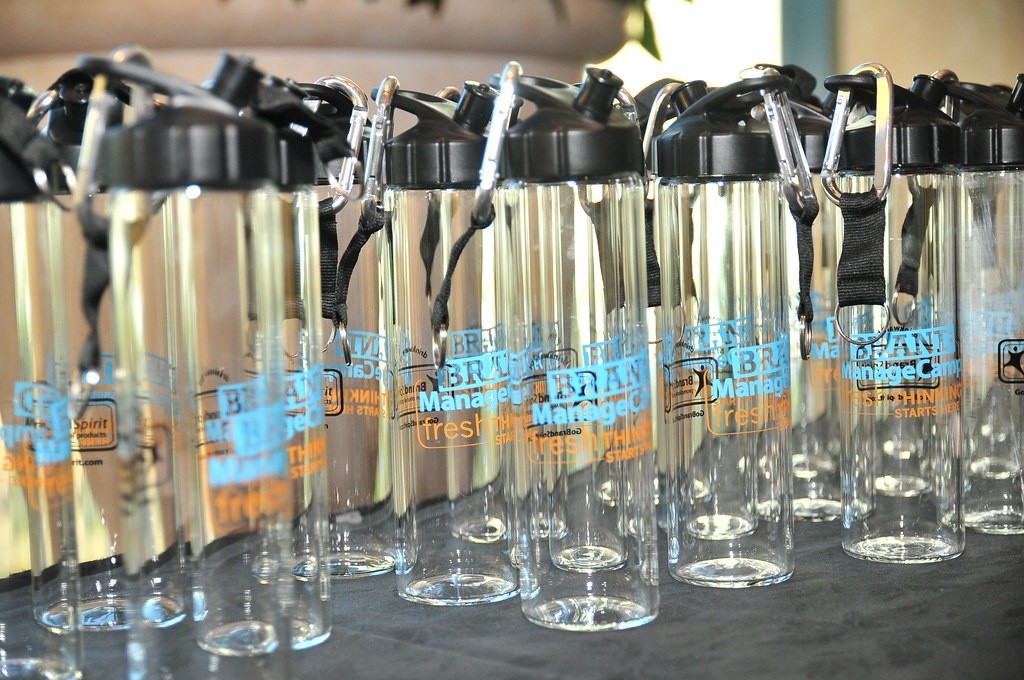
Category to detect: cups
[0,59,1024,680]
